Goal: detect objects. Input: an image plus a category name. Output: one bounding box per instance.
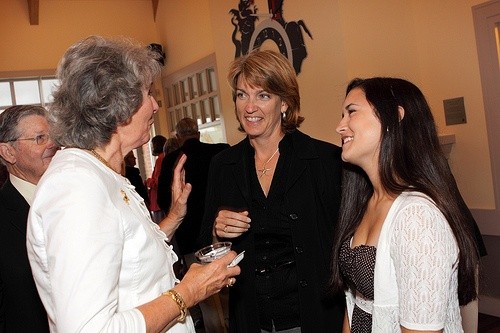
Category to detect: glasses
[5,133,55,145]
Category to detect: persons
[125,118,232,333]
[26,36,241,333]
[196,48,342,333]
[0,104,58,332]
[330,77,487,333]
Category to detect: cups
[195,242,232,264]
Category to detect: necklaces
[92,148,121,174]
[256,147,279,177]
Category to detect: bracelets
[163,289,188,322]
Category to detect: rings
[229,277,234,287]
[223,226,227,232]
